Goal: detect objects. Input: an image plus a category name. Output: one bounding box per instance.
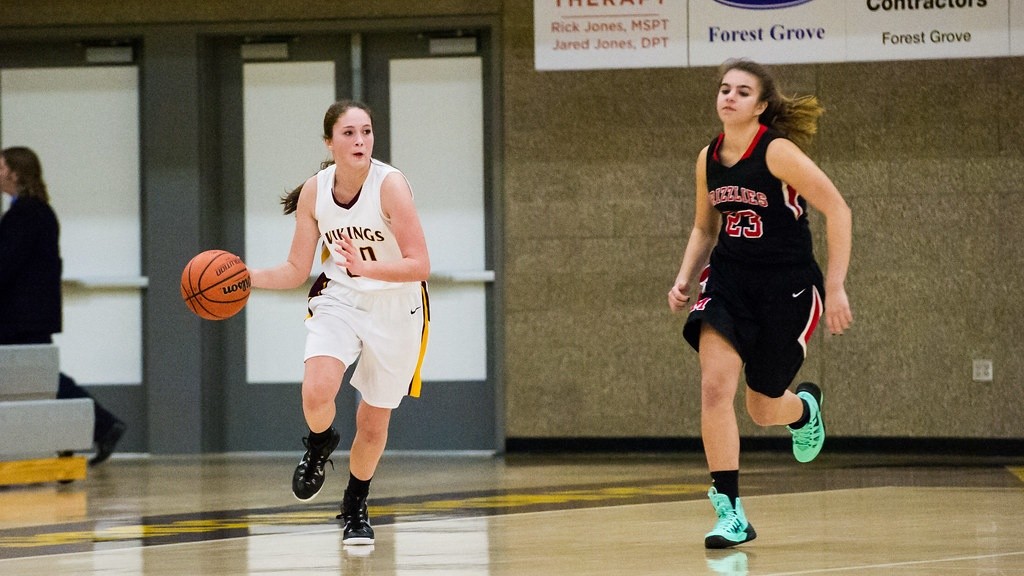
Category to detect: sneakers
[336,490,375,545]
[292,427,340,503]
[785,382,826,464]
[705,486,757,549]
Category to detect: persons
[0,147,126,466]
[237,100,431,546]
[668,61,853,548]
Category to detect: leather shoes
[90,419,128,467]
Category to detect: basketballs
[179,248,253,322]
